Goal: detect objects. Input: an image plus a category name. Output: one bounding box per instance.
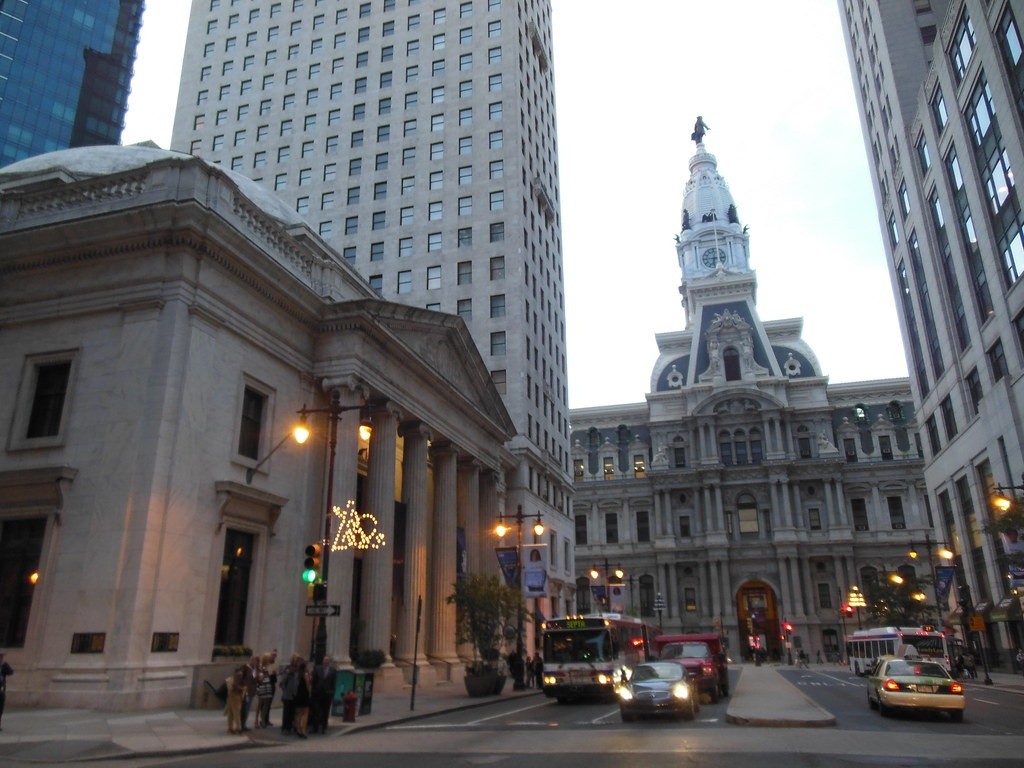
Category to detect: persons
[693,117,709,144]
[497,649,543,691]
[794,648,843,669]
[1016,649,1024,667]
[0,651,14,730]
[727,203,740,224]
[951,649,978,682]
[680,209,690,231]
[223,651,336,740]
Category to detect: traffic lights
[845,605,854,617]
[783,621,792,636]
[303,543,325,585]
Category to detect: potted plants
[445,570,535,699]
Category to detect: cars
[615,660,699,722]
[866,644,965,722]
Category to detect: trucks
[648,632,732,703]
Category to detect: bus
[540,613,663,703]
[848,627,952,675]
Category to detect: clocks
[701,247,728,270]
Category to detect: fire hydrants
[342,689,358,724]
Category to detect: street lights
[294,391,377,731]
[590,557,624,614]
[909,533,954,628]
[849,585,867,630]
[878,566,905,627]
[494,502,544,691]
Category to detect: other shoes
[255,720,274,729]
[309,728,326,734]
[281,729,294,736]
[227,726,252,735]
[297,733,308,739]
